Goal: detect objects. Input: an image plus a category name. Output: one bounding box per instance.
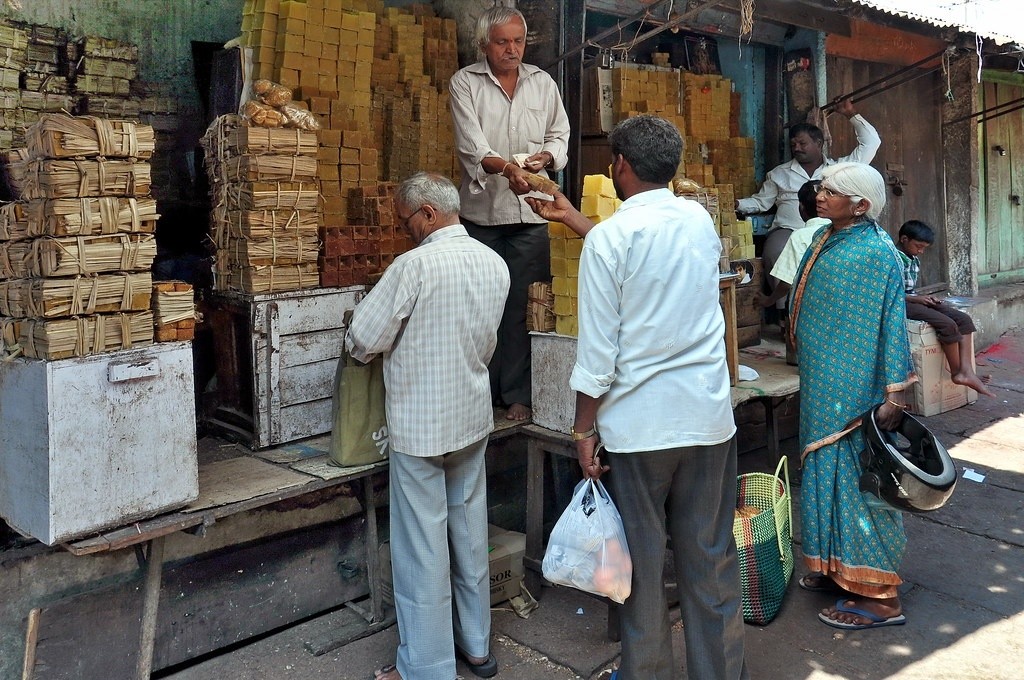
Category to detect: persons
[449,6,571,426]
[734,94,881,343]
[731,261,751,284]
[753,179,832,370]
[787,161,919,629]
[524,111,750,680]
[342,171,511,680]
[895,219,996,399]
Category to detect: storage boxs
[896,312,980,415]
[379,522,526,609]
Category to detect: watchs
[570,426,596,446]
[541,150,552,168]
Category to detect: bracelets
[886,397,907,410]
[499,162,512,178]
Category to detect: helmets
[859,403,958,513]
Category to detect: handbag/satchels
[542,477,633,605]
[327,310,389,468]
[732,455,795,626]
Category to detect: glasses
[399,205,437,231]
[813,185,864,200]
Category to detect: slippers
[373,664,458,680]
[818,596,906,629]
[454,642,497,678]
[799,571,854,594]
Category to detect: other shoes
[598,669,617,680]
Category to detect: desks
[61,411,532,674]
[720,275,744,386]
[518,342,814,640]
